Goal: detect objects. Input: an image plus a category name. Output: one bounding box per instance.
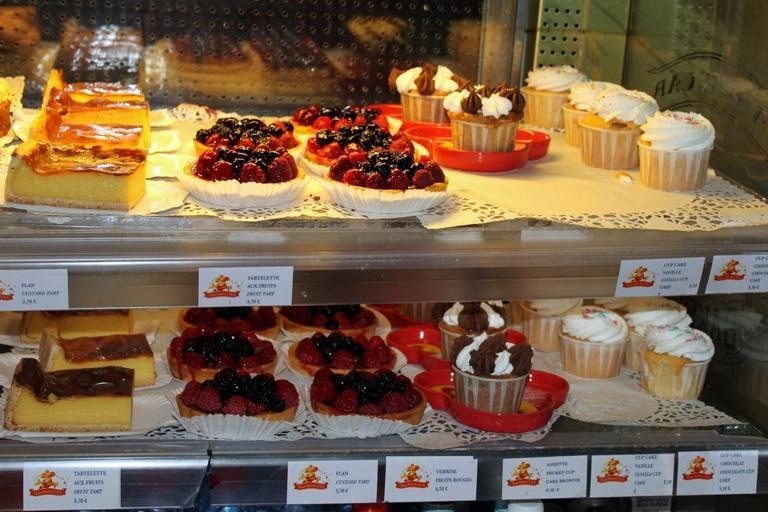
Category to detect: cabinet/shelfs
[0,0,767,511]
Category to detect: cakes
[299,122,430,176]
[449,328,534,415]
[278,306,378,336]
[19,308,133,344]
[577,89,660,171]
[558,305,631,380]
[42,67,146,111]
[443,80,527,152]
[320,147,455,214]
[193,115,306,166]
[4,139,149,212]
[37,333,156,388]
[174,144,311,207]
[519,298,584,353]
[287,103,403,146]
[177,306,281,339]
[165,326,280,383]
[46,86,152,148]
[636,108,716,192]
[175,367,300,424]
[562,79,627,148]
[308,367,427,427]
[26,107,145,150]
[288,330,397,377]
[4,358,135,432]
[636,324,716,403]
[519,63,589,132]
[438,300,507,362]
[395,62,466,124]
[618,298,694,372]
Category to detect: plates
[530,131,551,160]
[525,370,570,409]
[404,128,536,159]
[420,347,451,371]
[505,329,528,345]
[429,139,530,172]
[446,386,556,432]
[386,328,441,364]
[413,368,456,410]
